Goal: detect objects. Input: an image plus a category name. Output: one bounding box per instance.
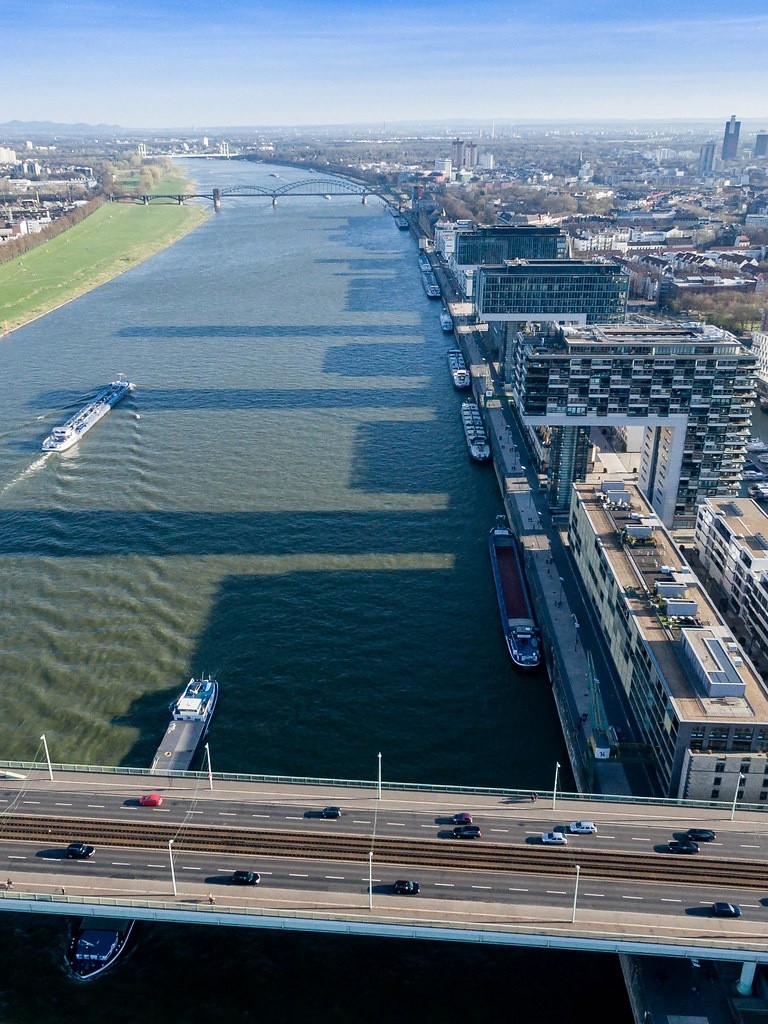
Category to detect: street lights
[552,761,561,810]
[572,865,582,924]
[368,852,374,909]
[40,734,54,781]
[731,772,745,822]
[378,752,382,800]
[205,743,213,790]
[168,839,178,897]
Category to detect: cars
[323,806,343,818]
[231,871,262,884]
[540,821,597,848]
[666,828,744,920]
[394,880,422,895]
[67,842,96,859]
[452,812,485,839]
[140,794,164,807]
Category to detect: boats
[390,206,442,298]
[461,396,494,462]
[446,349,470,391]
[440,308,454,332]
[41,380,134,454]
[150,675,219,769]
[58,917,139,985]
[489,514,544,668]
